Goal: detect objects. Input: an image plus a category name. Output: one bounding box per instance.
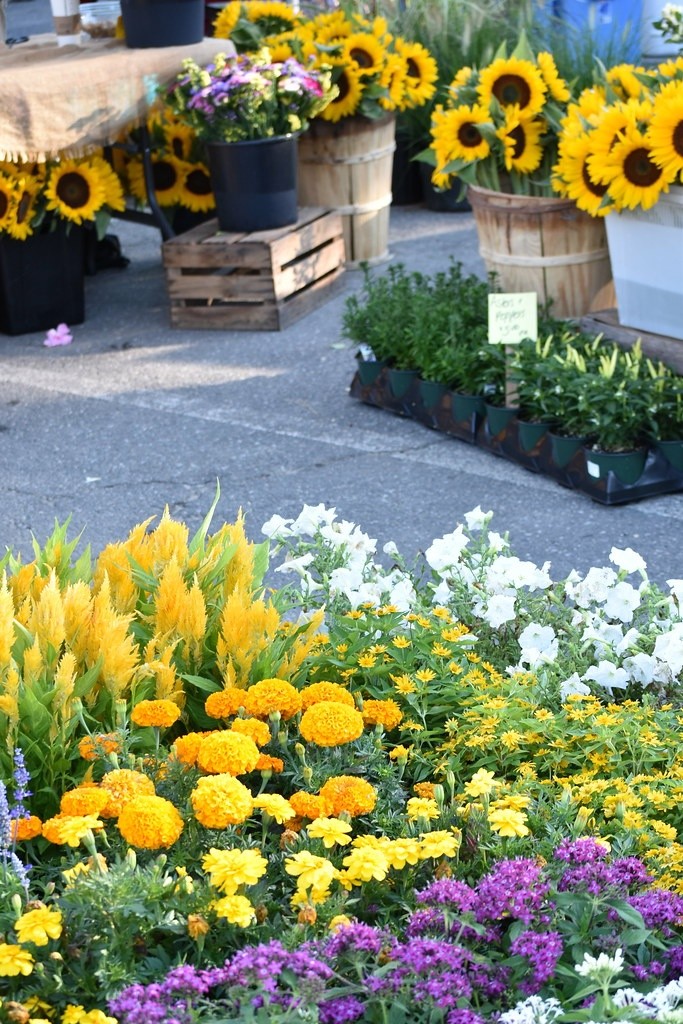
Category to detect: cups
[49,0,81,50]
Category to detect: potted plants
[339,257,683,507]
[333,0,614,213]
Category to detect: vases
[0,218,85,337]
[602,183,683,343]
[296,108,397,267]
[463,181,618,319]
[160,206,222,275]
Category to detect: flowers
[107,112,214,210]
[157,47,339,143]
[210,0,438,122]
[412,53,578,217]
[549,59,683,221]
[0,145,124,242]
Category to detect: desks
[0,30,238,161]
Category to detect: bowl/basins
[80,2,122,38]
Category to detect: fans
[201,133,299,232]
